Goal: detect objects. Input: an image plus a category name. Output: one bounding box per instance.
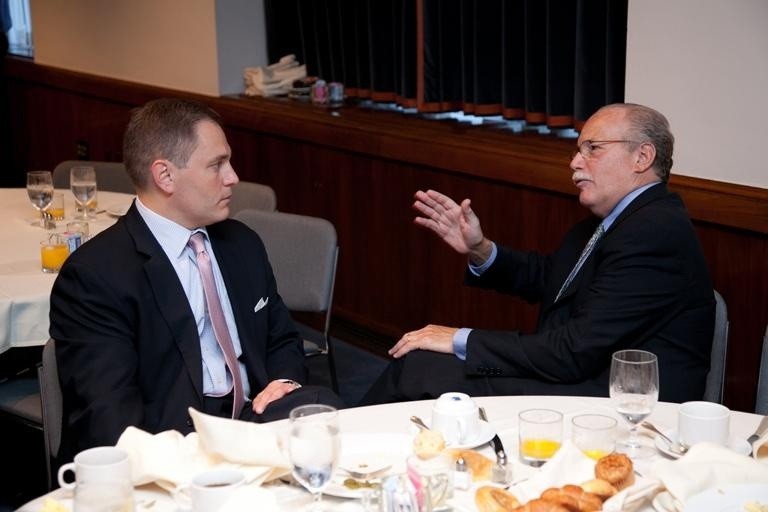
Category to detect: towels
[243,54,307,99]
[600,439,766,512]
[115,406,295,485]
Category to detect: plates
[505,441,655,510]
[435,417,495,451]
[654,425,754,460]
[109,203,134,214]
[59,492,162,512]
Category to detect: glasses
[569,139,635,159]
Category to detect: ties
[551,225,604,305]
[189,232,245,421]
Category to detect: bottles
[61,231,81,252]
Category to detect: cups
[48,194,65,222]
[289,404,343,512]
[42,241,70,272]
[176,469,248,512]
[50,232,80,252]
[680,400,730,448]
[572,417,617,460]
[72,197,97,213]
[68,222,89,238]
[520,409,564,468]
[428,392,477,445]
[58,446,136,498]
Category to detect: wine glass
[71,166,102,221]
[609,350,660,447]
[27,170,55,227]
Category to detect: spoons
[643,419,689,453]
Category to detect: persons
[387,102,717,404]
[49,98,350,456]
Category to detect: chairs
[228,180,277,223]
[755,326,768,416]
[1,340,47,431]
[42,339,69,493]
[702,289,729,405]
[51,160,137,196]
[233,208,341,392]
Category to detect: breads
[583,481,617,502]
[595,453,635,489]
[513,485,601,512]
[476,486,521,512]
